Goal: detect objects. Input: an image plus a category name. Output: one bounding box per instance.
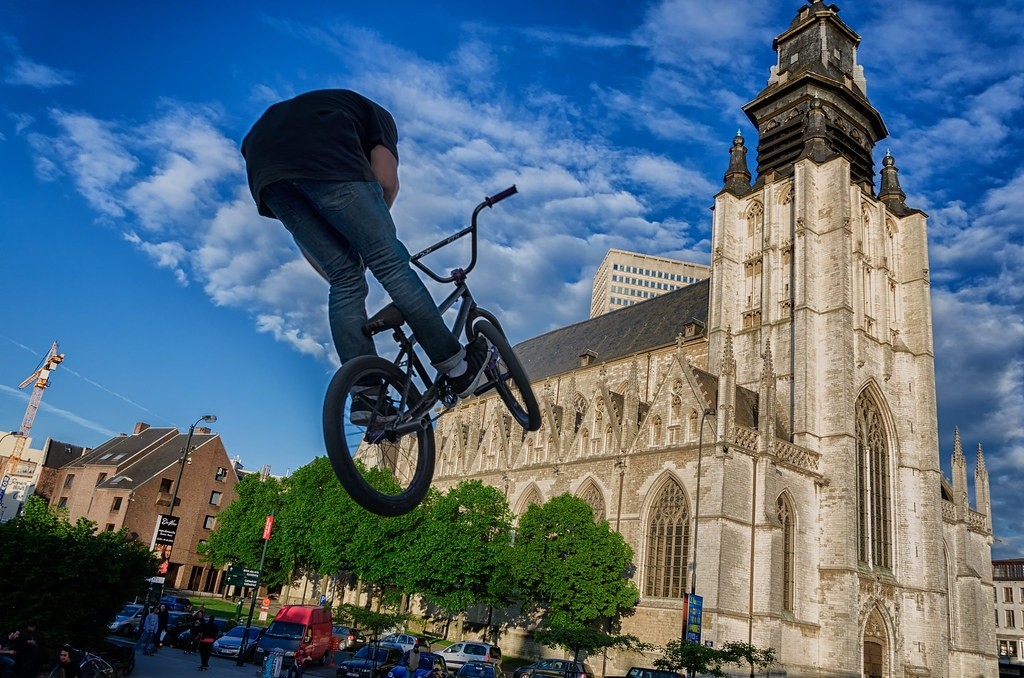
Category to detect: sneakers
[350,395,400,425]
[448,337,491,399]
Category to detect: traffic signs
[224,565,260,589]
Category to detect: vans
[254,605,333,668]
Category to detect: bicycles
[323,185,542,518]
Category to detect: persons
[240,88,494,425]
[0,603,446,678]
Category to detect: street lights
[136,415,217,640]
[691,408,716,593]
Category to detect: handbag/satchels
[160,630,167,641]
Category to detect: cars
[157,594,191,611]
[210,625,268,661]
[452,662,506,678]
[336,646,404,678]
[371,633,432,654]
[332,624,367,652]
[106,604,155,636]
[386,650,449,678]
[513,658,595,678]
[432,642,503,670]
[176,618,238,652]
[135,611,196,645]
